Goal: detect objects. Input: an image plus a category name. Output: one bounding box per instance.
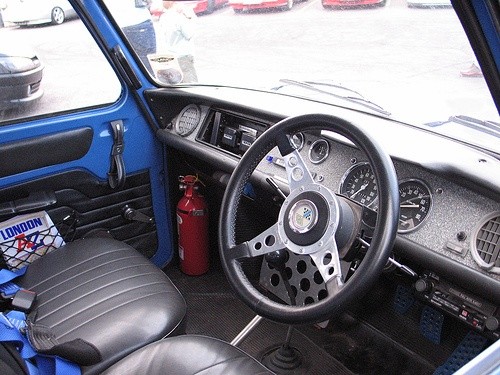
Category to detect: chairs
[0,237,187,375]
[101,335,277,375]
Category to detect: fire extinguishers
[176,175,209,276]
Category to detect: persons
[102,0,198,84]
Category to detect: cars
[0,51,43,111]
[228,1,309,13]
[146,0,229,18]
[321,0,387,8]
[406,1,452,8]
[2,0,78,28]
